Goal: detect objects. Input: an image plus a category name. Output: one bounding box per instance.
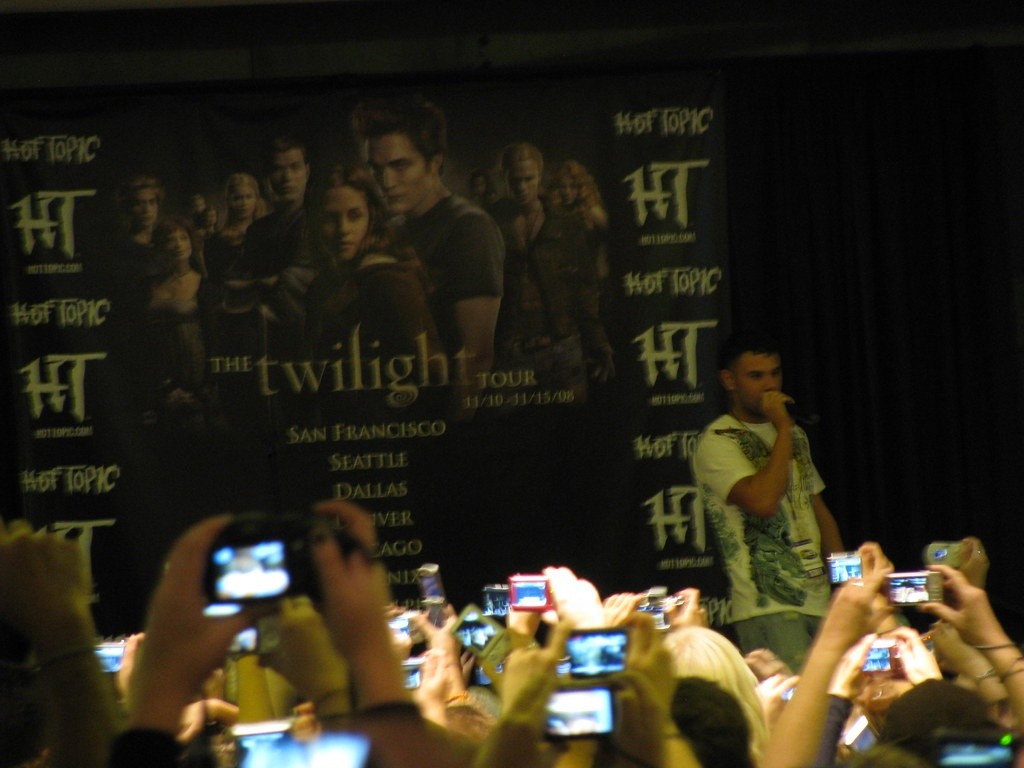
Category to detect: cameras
[203,516,686,768]
[782,544,1024,768]
[97,644,125,674]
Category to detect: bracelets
[312,685,349,705]
[663,732,683,741]
[999,669,1023,679]
[975,667,997,682]
[445,691,469,707]
[29,644,98,677]
[362,701,421,719]
[977,642,1018,651]
[1000,657,1024,684]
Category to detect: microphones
[785,401,819,425]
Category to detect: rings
[853,582,863,587]
[163,561,171,576]
[367,546,382,561]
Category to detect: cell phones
[417,564,448,627]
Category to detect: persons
[693,334,848,673]
[0,500,1024,768]
[118,91,614,421]
